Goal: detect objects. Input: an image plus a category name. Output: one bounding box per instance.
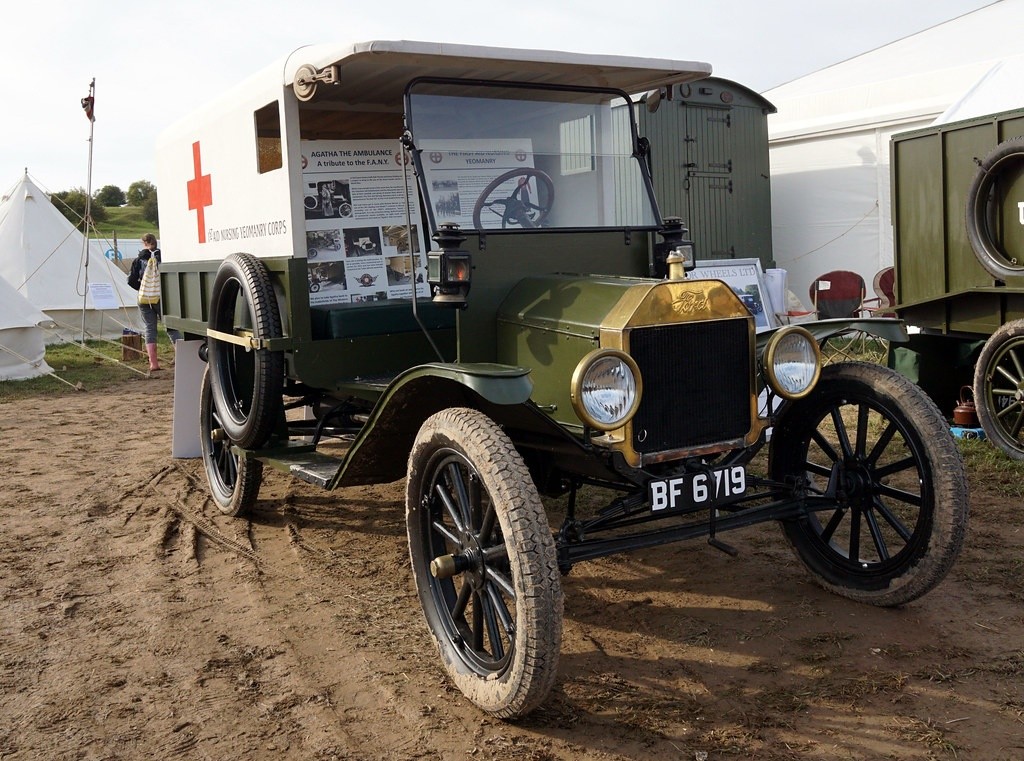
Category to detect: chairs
[810,270,872,352]
[872,266,897,317]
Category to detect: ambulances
[152,39,970,720]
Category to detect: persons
[127,232,184,370]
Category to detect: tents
[0,167,137,387]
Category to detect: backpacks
[137,249,161,306]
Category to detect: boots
[145,343,160,371]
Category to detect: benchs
[304,294,459,342]
